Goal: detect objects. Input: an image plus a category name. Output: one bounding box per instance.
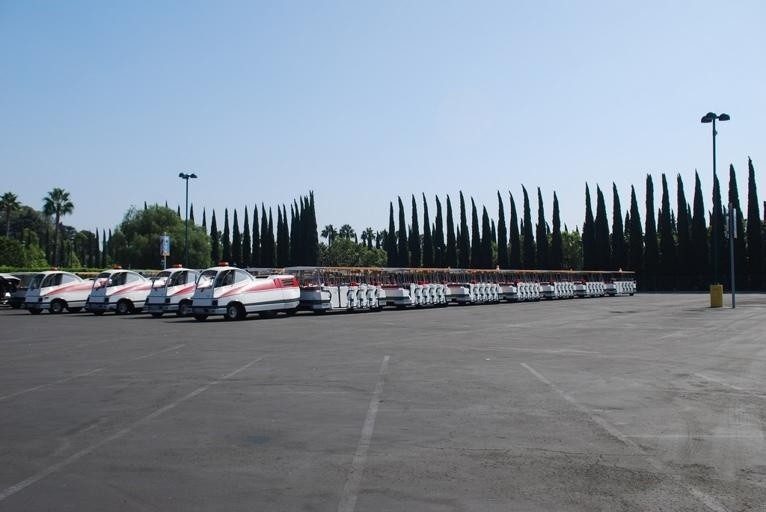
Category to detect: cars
[188,261,300,322]
[21,266,96,315]
[141,262,214,321]
[0,273,20,306]
[83,264,163,316]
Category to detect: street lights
[176,172,198,266]
[699,111,731,309]
